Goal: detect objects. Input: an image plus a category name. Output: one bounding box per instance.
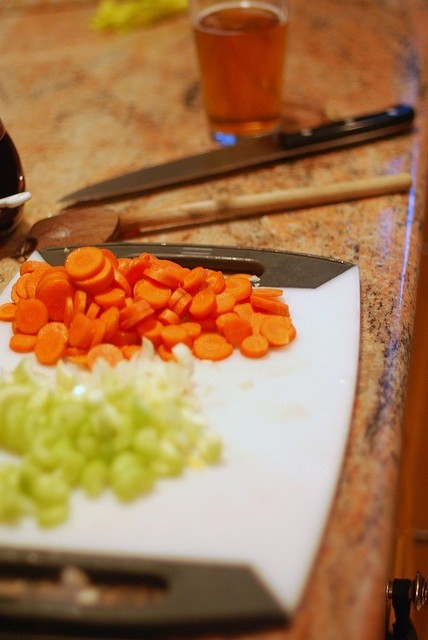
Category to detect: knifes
[55,104,418,202]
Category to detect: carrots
[0,248,297,362]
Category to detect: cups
[194,5,290,137]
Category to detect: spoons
[12,169,411,252]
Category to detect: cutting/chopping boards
[2,243,358,623]
